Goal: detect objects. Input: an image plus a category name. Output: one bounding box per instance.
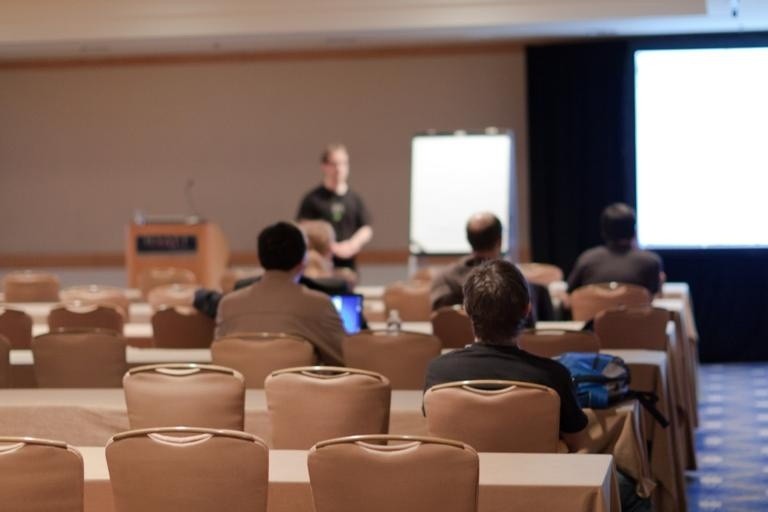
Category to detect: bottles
[386,309,403,338]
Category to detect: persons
[419,258,592,454]
[558,201,665,333]
[214,142,376,376]
[426,211,536,335]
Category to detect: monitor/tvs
[328,293,362,332]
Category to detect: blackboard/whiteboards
[410,133,513,254]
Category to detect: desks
[0,383,656,499]
[72,446,624,512]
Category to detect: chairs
[422,380,561,454]
[0,436,84,512]
[307,434,480,512]
[264,366,391,448]
[121,363,245,434]
[104,426,268,512]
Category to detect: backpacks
[553,352,668,429]
